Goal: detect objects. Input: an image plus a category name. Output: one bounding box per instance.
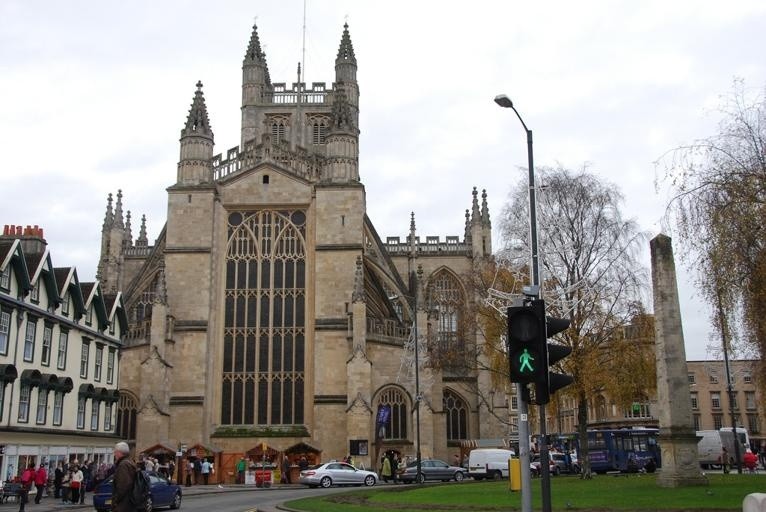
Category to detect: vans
[532,452,581,477]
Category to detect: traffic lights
[534,298,575,406]
[507,306,546,382]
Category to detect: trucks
[468,449,538,481]
[696,430,746,469]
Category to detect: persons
[743,447,759,471]
[453,455,459,467]
[282,455,293,484]
[392,453,401,484]
[342,455,348,462]
[348,456,354,463]
[298,456,308,470]
[236,457,246,482]
[381,455,391,483]
[462,453,471,479]
[719,446,730,474]
[20,441,214,504]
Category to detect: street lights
[389,293,421,484]
[494,93,552,510]
[700,253,743,475]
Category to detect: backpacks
[129,470,150,508]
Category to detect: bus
[587,427,662,475]
[530,432,579,457]
[719,427,753,468]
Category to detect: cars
[570,453,582,465]
[394,459,468,484]
[93,470,182,512]
[299,461,379,488]
